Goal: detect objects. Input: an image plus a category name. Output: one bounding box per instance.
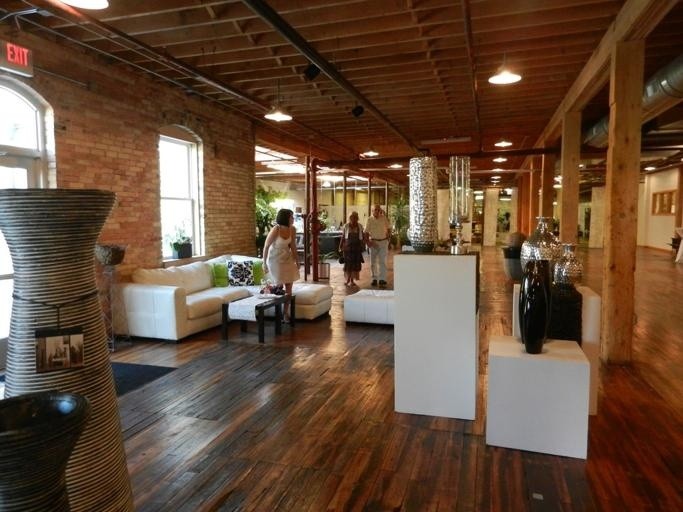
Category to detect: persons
[365,204,392,286]
[503,212,511,233]
[339,211,366,286]
[263,209,300,324]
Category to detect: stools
[345,289,393,325]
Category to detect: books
[258,293,276,299]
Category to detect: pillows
[254,261,264,285]
[228,261,254,286]
[213,263,228,287]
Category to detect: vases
[502,216,583,352]
[0,188,133,512]
[0,391,92,512]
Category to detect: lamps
[487,53,522,85]
[352,106,364,118]
[264,81,293,122]
[303,65,320,81]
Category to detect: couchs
[110,255,333,341]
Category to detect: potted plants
[170,228,192,259]
[92,241,128,265]
